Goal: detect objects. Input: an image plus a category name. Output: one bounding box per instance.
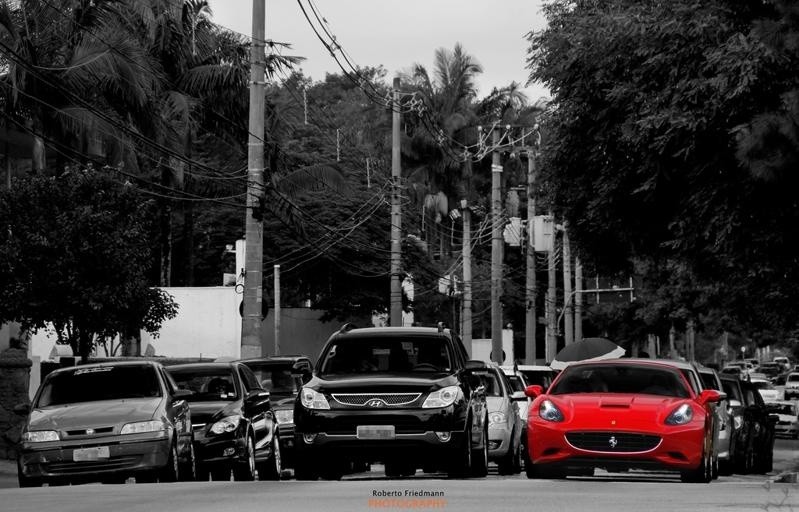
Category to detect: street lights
[741,346,745,361]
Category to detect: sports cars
[524,360,720,483]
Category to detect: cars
[290,321,489,481]
[13,359,198,487]
[163,362,283,482]
[229,356,799,476]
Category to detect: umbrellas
[549,336,629,370]
